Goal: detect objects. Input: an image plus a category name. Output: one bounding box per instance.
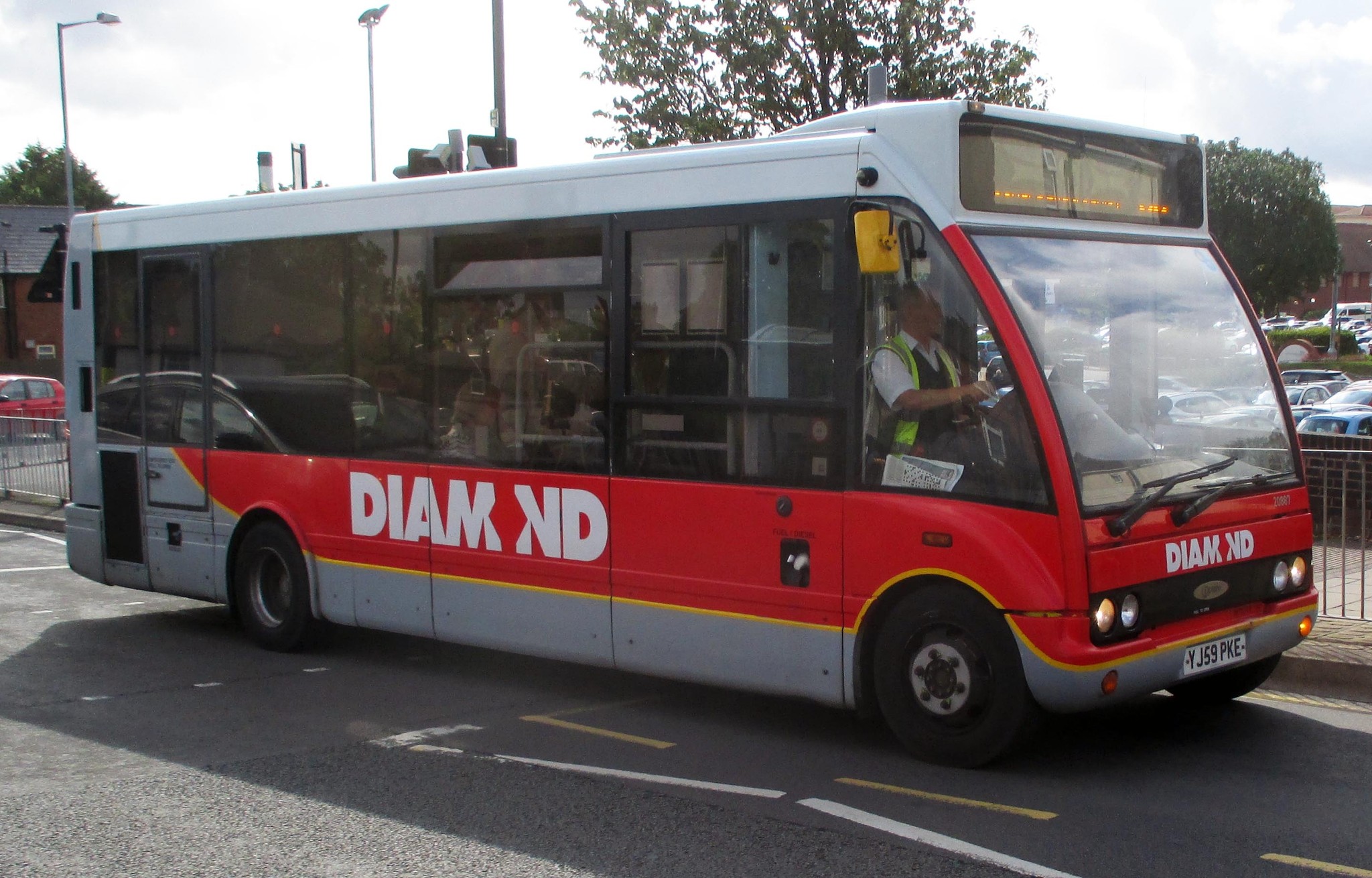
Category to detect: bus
[63,98,1321,771]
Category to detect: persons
[862,280,997,465]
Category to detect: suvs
[96,366,441,467]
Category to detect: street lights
[56,11,123,224]
[357,3,390,183]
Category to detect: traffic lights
[393,147,448,179]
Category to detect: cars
[972,300,1372,506]
[0,374,64,443]
